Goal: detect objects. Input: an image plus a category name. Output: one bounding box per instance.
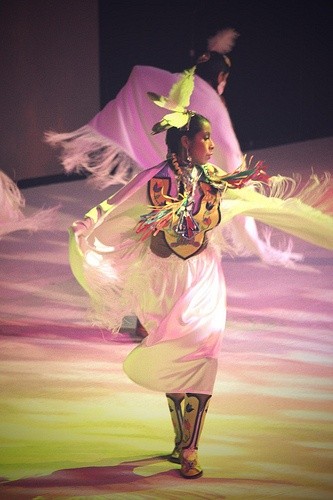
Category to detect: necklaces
[138,153,204,240]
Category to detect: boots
[166,395,185,462]
[178,392,212,479]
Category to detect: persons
[131,28,254,339]
[67,111,285,478]
